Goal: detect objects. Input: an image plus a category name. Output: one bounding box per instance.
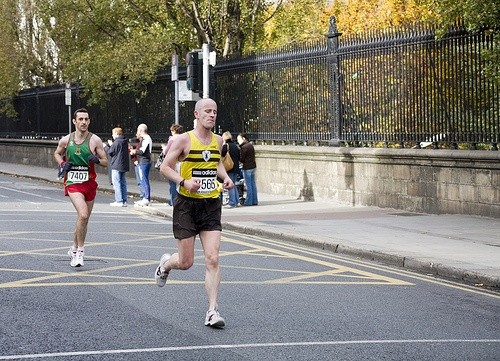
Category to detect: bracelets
[60,161,64,168]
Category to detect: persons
[57,123,153,206]
[217,130,258,209]
[54,108,109,266]
[154,98,235,328]
[160,125,185,206]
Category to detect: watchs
[180,178,185,186]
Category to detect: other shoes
[224,204,231,209]
[135,199,150,207]
[237,202,241,206]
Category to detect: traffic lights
[184,50,197,90]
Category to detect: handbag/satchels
[155,151,164,169]
[220,143,234,172]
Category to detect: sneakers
[109,201,127,208]
[68,246,85,267]
[204,309,226,329]
[155,253,171,287]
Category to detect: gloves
[88,155,100,165]
[61,161,72,172]
